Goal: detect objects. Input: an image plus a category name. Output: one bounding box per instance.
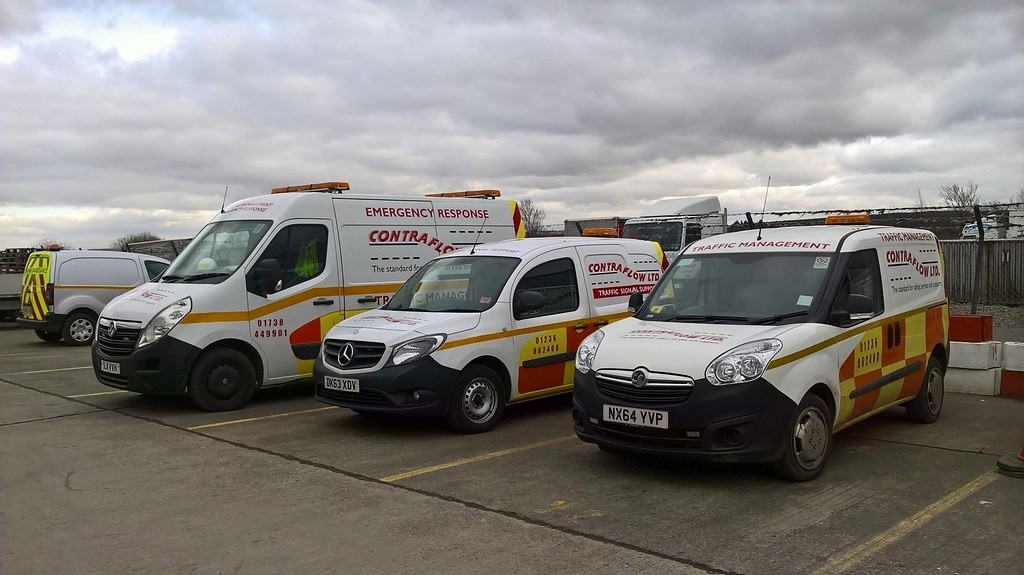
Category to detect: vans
[16,244,174,345]
[573,175,953,478]
[311,219,678,434]
[90,182,527,412]
